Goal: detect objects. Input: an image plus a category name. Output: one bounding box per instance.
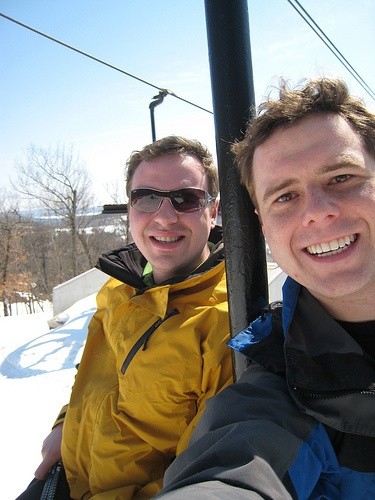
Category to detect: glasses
[130,188,215,213]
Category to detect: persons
[15,135,235,500]
[146,76,375,500]
